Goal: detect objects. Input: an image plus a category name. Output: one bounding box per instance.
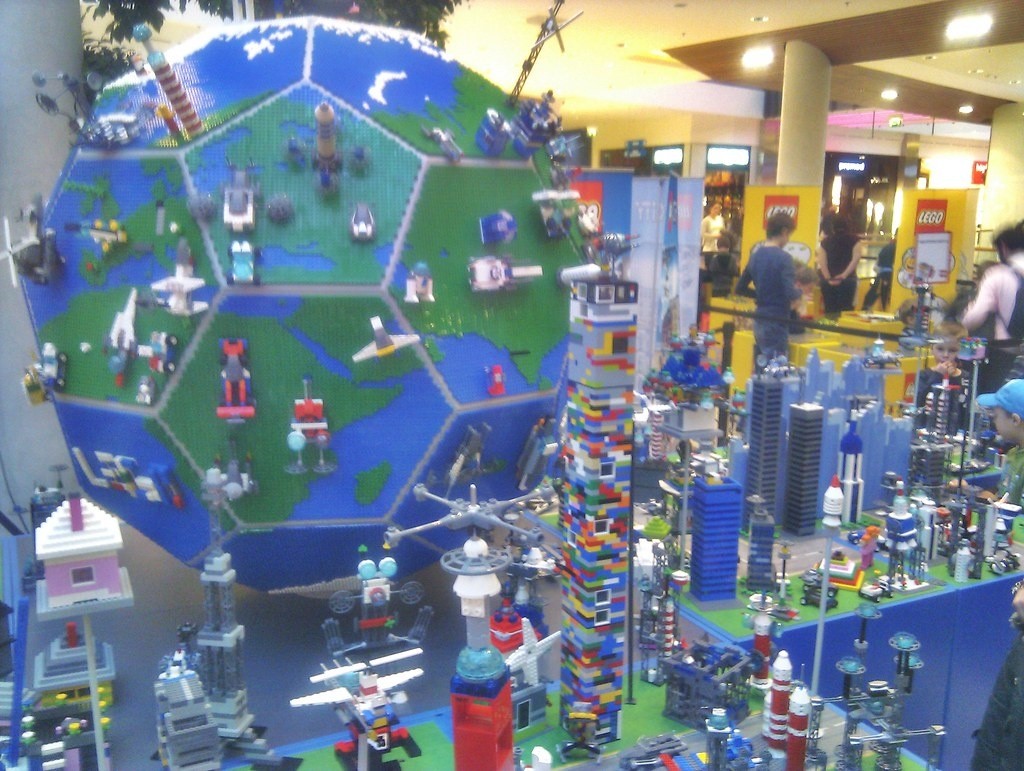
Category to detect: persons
[961,219,1024,393]
[863,226,899,312]
[817,204,862,312]
[734,212,803,359]
[916,321,972,430]
[701,201,737,298]
[976,379,1024,508]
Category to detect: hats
[977,378,1024,421]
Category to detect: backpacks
[997,260,1024,340]
